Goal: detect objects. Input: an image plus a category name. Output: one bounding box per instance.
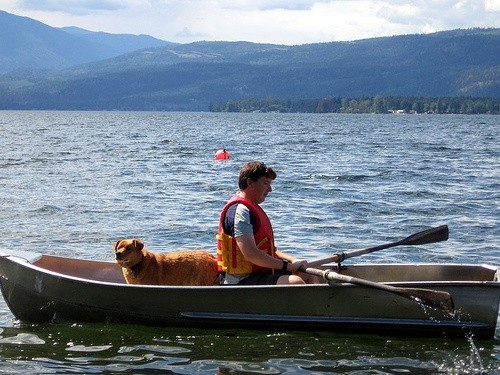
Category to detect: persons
[219,161,306,284]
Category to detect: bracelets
[283,259,288,273]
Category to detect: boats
[0,246,500,342]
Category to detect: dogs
[112,238,219,286]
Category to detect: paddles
[297,264,455,313]
[306,224,449,264]
